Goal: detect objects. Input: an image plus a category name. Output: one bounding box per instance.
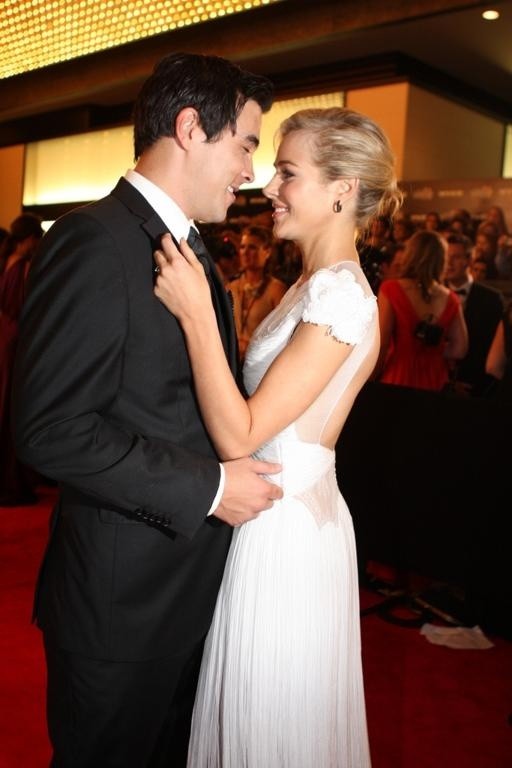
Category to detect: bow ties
[454,289,467,295]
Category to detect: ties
[187,228,229,362]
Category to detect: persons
[0,213,46,505]
[154,106,401,768]
[334,207,512,638]
[199,213,304,359]
[2,58,283,768]
